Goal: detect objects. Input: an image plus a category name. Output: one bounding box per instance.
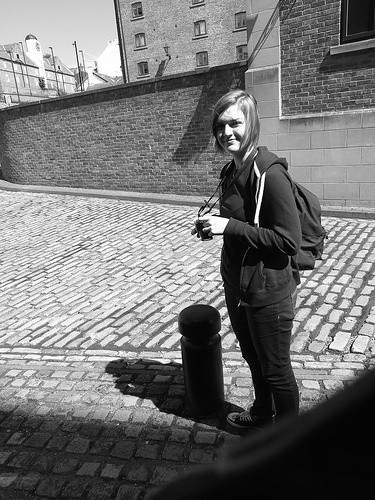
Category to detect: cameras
[196,223,213,241]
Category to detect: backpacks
[292,180,328,270]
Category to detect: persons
[190,90,303,432]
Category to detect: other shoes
[226,410,272,431]
